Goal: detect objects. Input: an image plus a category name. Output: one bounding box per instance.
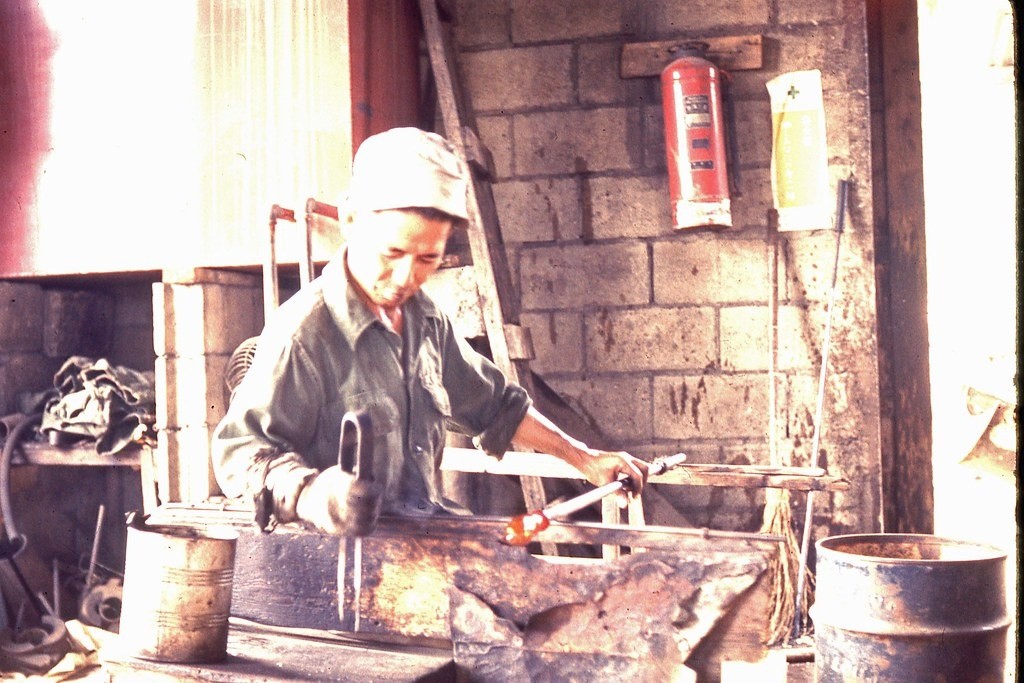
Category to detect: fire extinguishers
[663,40,742,233]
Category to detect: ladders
[420,0,588,558]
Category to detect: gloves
[294,466,383,539]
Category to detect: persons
[210,126,648,537]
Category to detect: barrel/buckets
[807,532,1012,683]
[118,522,240,665]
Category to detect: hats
[353,125,468,222]
